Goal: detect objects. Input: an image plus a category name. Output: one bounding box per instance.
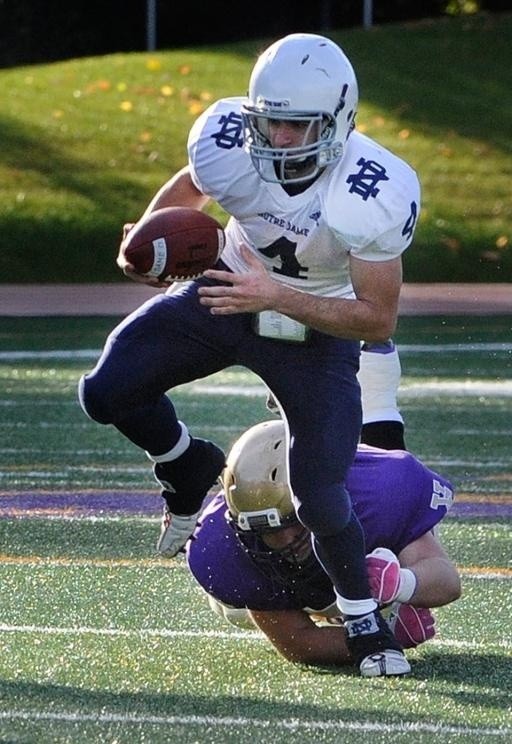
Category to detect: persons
[185,335,464,667]
[77,32,421,678]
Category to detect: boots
[359,340,404,424]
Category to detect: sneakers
[343,610,411,676]
[153,438,225,559]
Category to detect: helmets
[223,419,298,532]
[248,33,358,147]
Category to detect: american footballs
[121,206,226,282]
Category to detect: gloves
[390,601,436,649]
[365,548,418,603]
